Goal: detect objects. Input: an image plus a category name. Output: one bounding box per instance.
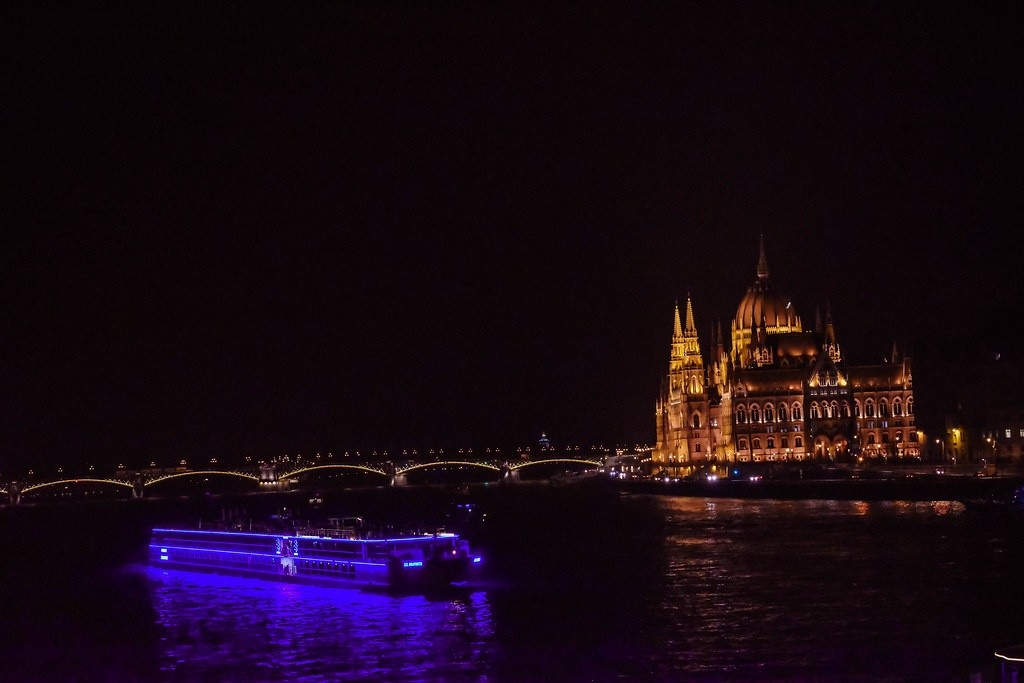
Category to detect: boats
[149,507,485,590]
